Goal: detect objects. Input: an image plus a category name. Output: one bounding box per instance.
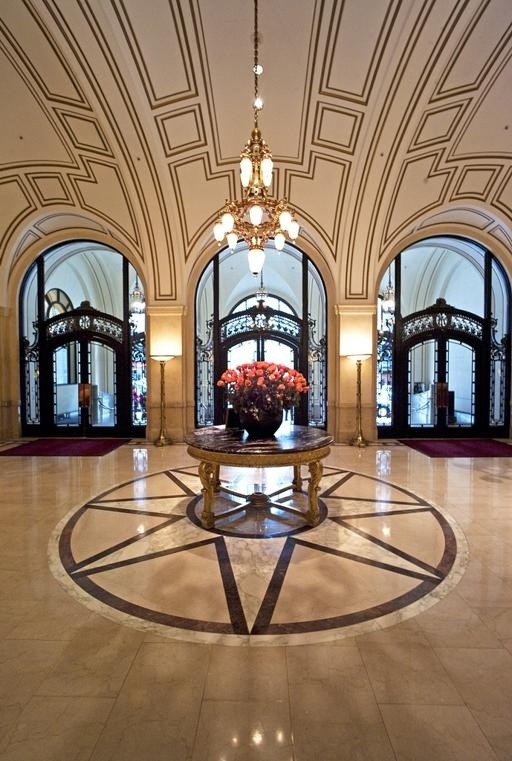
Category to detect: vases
[239,409,284,437]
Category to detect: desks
[183,424,334,528]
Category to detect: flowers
[216,359,311,414]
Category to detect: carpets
[0,437,132,458]
[397,438,512,458]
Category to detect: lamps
[213,1,301,284]
[150,356,177,446]
[344,353,374,449]
